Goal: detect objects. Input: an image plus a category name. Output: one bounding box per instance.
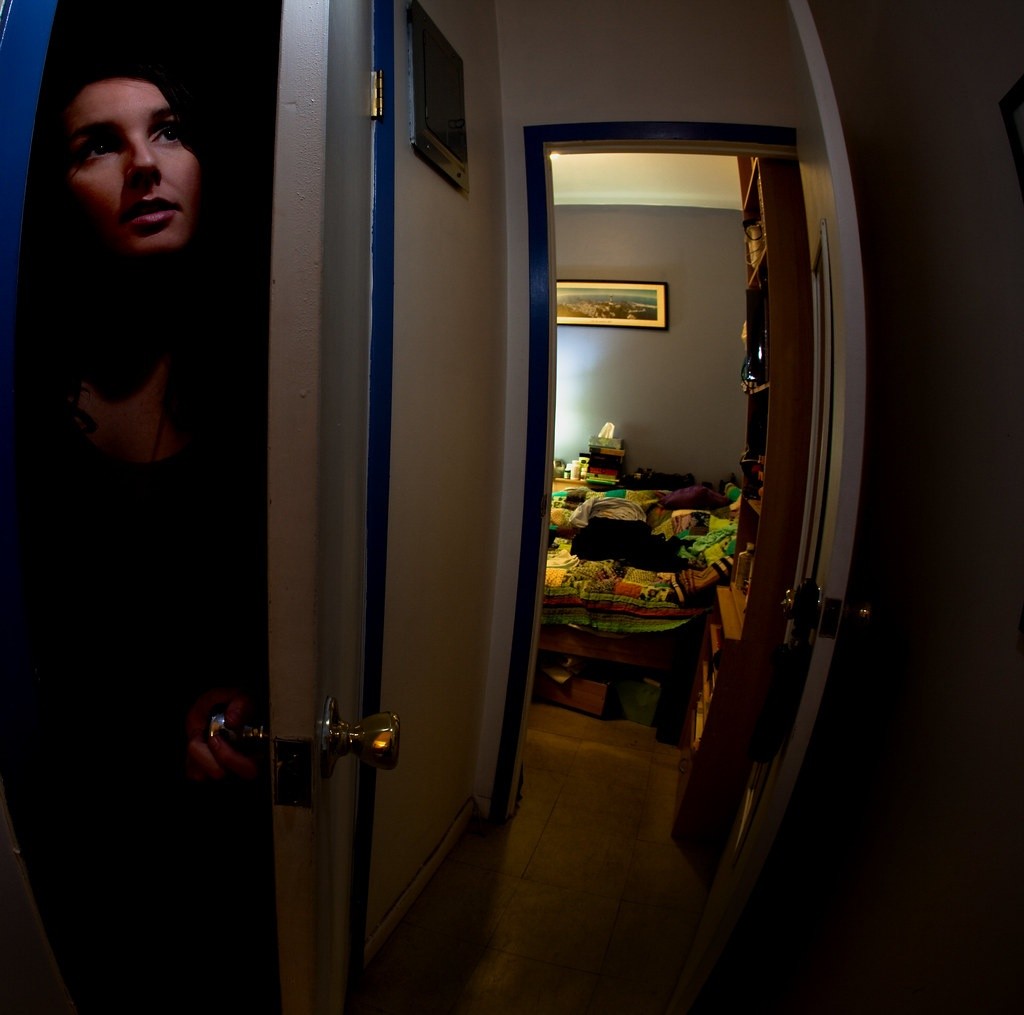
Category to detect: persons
[1,52,282,1015]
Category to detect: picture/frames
[556,279,670,332]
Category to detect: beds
[537,483,742,670]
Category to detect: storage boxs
[612,676,662,727]
[537,673,618,718]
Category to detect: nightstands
[553,478,615,492]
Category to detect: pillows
[656,486,733,511]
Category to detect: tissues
[589,421,624,449]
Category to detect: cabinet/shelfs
[672,586,780,839]
[730,154,813,639]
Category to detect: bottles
[580,460,588,480]
[735,543,754,590]
[564,470,571,479]
[571,460,580,479]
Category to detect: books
[585,445,625,485]
